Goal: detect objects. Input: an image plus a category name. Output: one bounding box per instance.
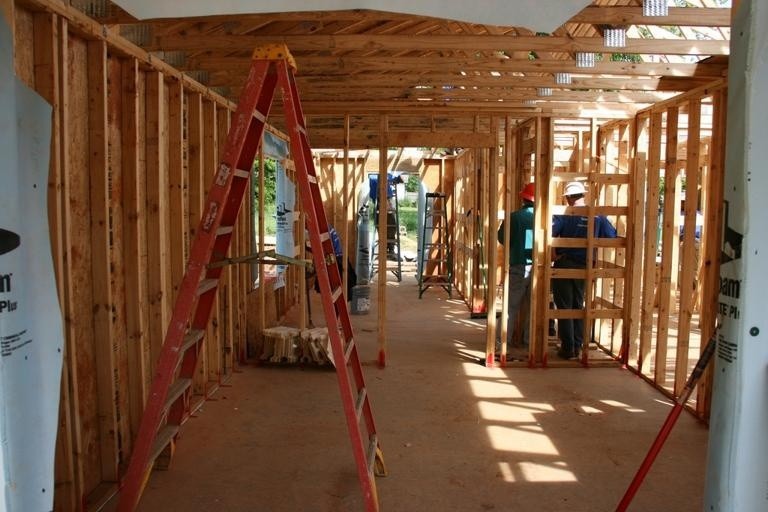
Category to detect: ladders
[370,177,402,282]
[419,193,452,299]
[116,43,387,512]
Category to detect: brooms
[471,210,503,318]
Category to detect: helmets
[562,182,588,196]
[519,183,535,202]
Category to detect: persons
[494,182,536,362]
[552,181,617,360]
[373,198,403,261]
